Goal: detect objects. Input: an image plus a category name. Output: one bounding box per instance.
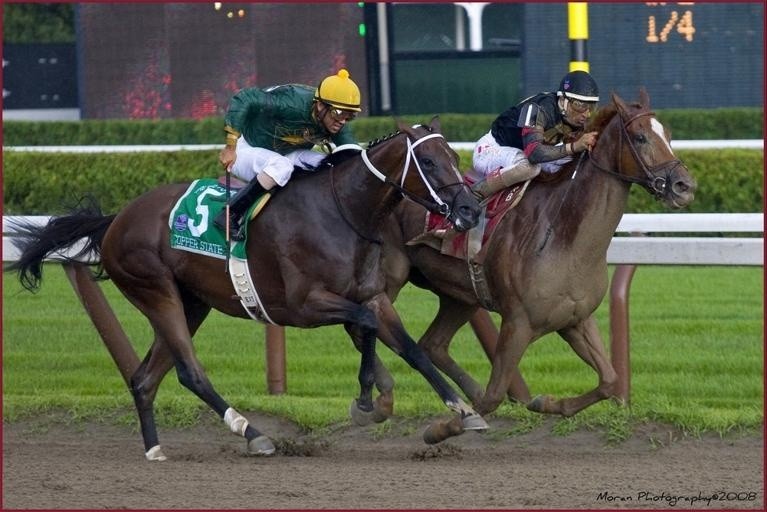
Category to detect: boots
[212,173,269,242]
[468,165,507,205]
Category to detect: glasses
[324,103,357,122]
[569,98,597,113]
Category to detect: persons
[212,69,361,240]
[469,70,600,204]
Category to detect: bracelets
[570,142,578,154]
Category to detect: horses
[343,86,698,423]
[3,115,490,461]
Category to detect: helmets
[313,68,362,113]
[556,70,600,103]
